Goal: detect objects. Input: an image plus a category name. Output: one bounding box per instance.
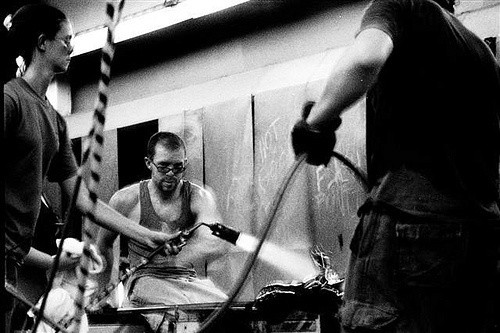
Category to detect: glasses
[149,160,186,175]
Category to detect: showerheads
[210,223,241,245]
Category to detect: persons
[0,3,183,333]
[290,0,500,333]
[89,132,232,333]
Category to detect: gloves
[290,100,342,167]
